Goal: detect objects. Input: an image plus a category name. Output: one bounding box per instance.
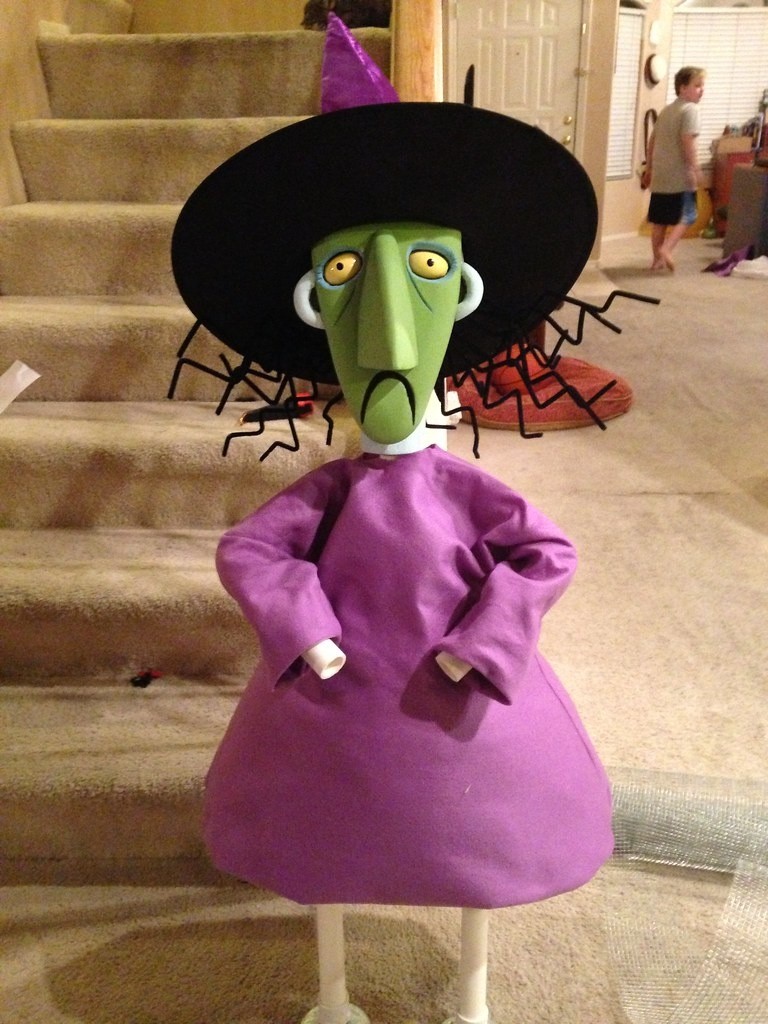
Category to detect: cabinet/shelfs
[714,149,768,238]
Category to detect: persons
[643,68,705,273]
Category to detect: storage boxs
[712,137,752,153]
